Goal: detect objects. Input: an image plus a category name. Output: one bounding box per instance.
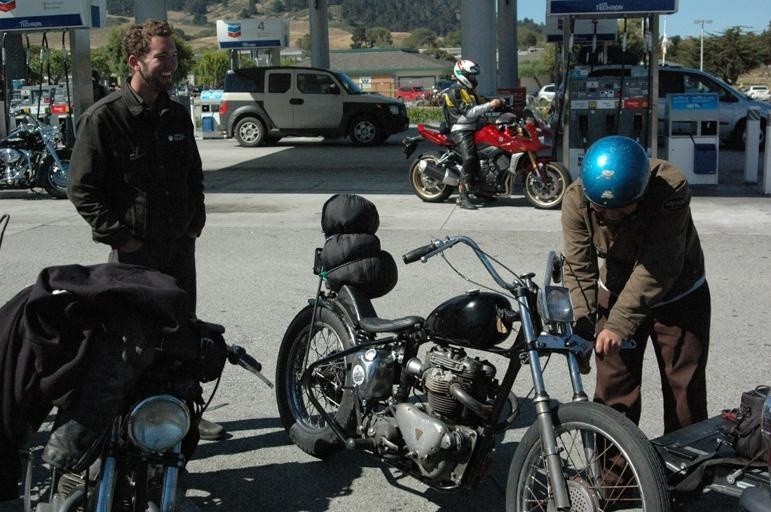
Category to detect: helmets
[579,134,651,209]
[453,59,480,89]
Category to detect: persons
[440,57,511,210]
[91,68,114,105]
[64,17,227,441]
[558,131,714,511]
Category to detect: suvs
[218,66,409,147]
[533,82,557,106]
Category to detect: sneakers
[195,416,225,440]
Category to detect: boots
[456,176,478,210]
[475,192,497,201]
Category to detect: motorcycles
[0,279,274,512]
[402,97,573,209]
[275,234,673,512]
[0,110,78,198]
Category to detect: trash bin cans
[202,117,214,132]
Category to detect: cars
[394,84,433,101]
[562,63,771,149]
